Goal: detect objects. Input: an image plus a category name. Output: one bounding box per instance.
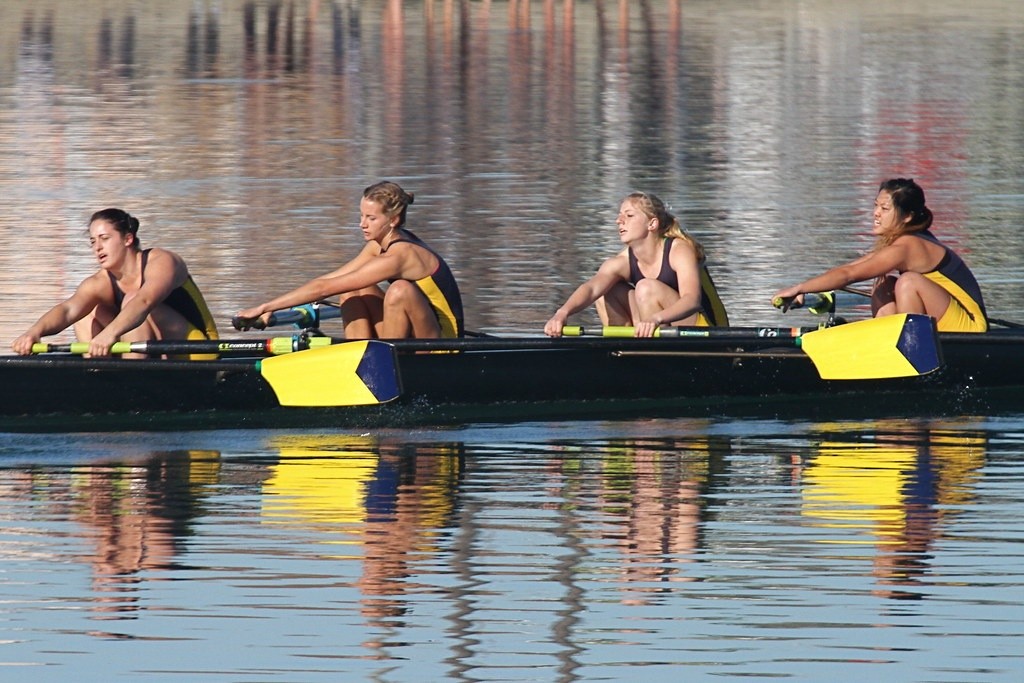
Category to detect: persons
[11,208,221,361]
[235,180,464,340]
[544,192,728,337]
[771,178,989,333]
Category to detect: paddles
[1,295,1024,408]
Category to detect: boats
[0,324,1024,435]
